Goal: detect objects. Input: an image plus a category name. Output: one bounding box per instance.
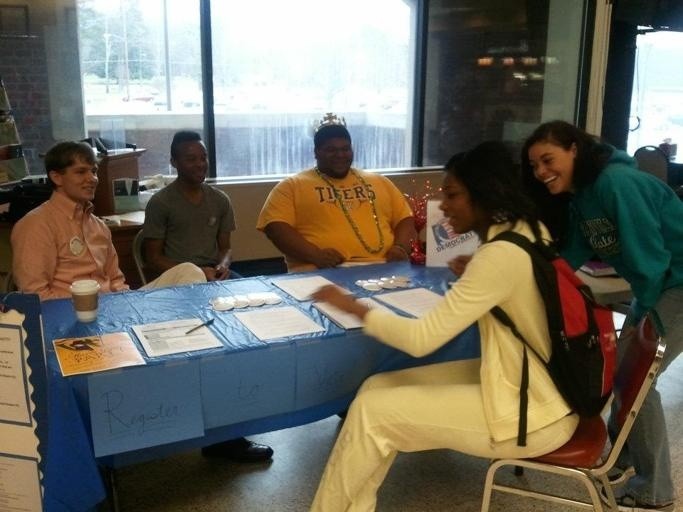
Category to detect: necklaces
[313,166,384,254]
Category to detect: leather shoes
[203,439,273,463]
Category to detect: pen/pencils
[185,318,215,334]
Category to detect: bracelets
[392,240,412,257]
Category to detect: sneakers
[599,460,674,511]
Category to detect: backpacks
[477,234,618,419]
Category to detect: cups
[70,279,100,323]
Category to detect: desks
[38,257,481,511]
[93,208,146,291]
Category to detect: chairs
[634,147,683,199]
[480,311,666,511]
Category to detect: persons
[254,122,418,274]
[142,128,247,282]
[308,146,582,512]
[9,140,274,463]
[443,119,683,511]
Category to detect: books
[579,261,617,278]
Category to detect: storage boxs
[91,153,143,217]
